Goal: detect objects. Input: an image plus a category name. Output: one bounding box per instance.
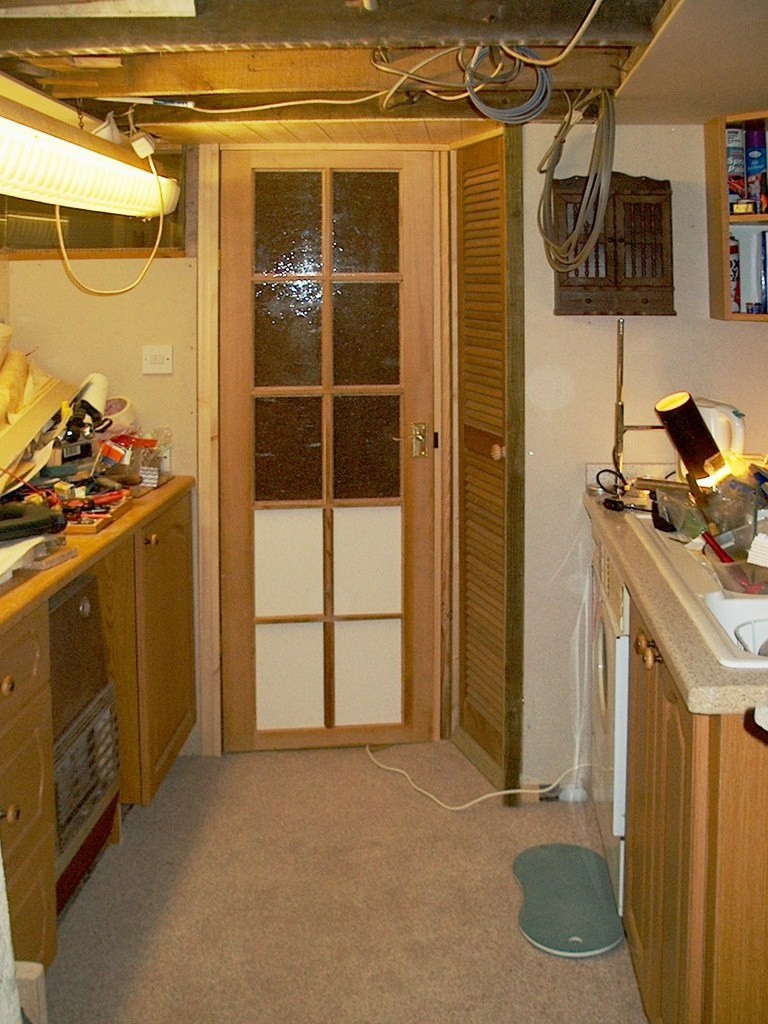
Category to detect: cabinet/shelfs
[88,491,200,806]
[0,597,54,974]
[703,111,768,322]
[622,598,768,1024]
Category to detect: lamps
[0,93,181,219]
[655,389,731,492]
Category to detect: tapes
[103,396,137,431]
